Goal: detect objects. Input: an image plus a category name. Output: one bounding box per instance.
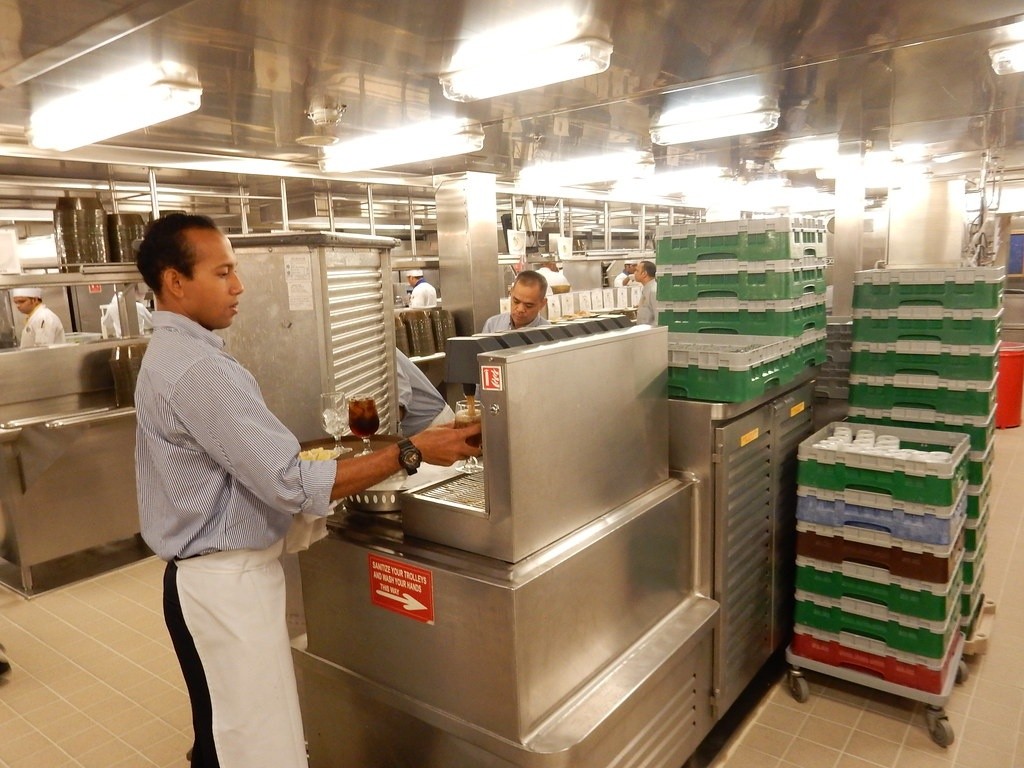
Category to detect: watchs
[397,438,422,476]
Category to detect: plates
[325,450,340,460]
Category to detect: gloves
[629,274,635,280]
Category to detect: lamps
[438,37,613,103]
[27,83,204,151]
[317,121,487,173]
[988,41,1024,75]
[649,110,781,146]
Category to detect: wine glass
[319,392,353,454]
[348,393,380,458]
[453,400,485,475]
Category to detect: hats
[12,287,41,299]
[624,259,637,265]
[406,267,423,277]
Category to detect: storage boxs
[655,217,1006,695]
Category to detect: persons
[613,253,638,287]
[105,281,152,359]
[535,257,572,296]
[473,270,552,401]
[396,346,455,439]
[133,210,482,768]
[634,261,657,325]
[12,273,66,349]
[402,269,437,309]
